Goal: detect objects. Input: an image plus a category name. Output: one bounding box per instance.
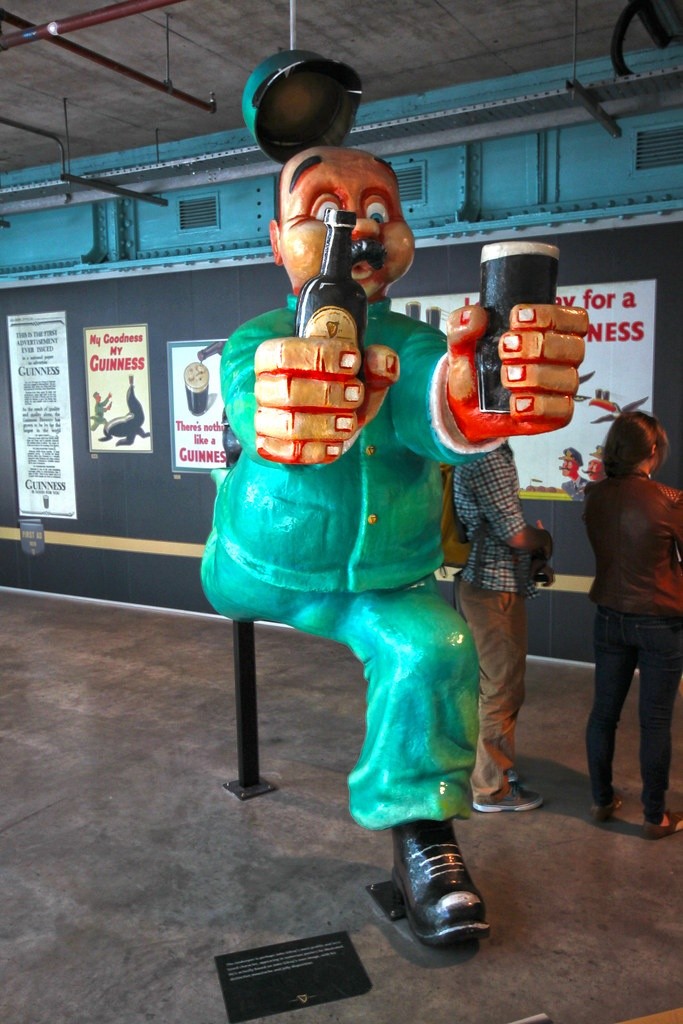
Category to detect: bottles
[294,211,364,381]
[198,342,224,361]
[406,301,421,319]
[426,306,441,326]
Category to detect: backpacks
[440,445,490,569]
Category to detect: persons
[452,441,554,812]
[585,411,683,839]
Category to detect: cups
[481,241,560,414]
[184,362,209,416]
[129,375,133,385]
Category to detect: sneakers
[504,769,519,782]
[473,782,544,813]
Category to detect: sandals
[640,809,683,839]
[591,793,622,822]
